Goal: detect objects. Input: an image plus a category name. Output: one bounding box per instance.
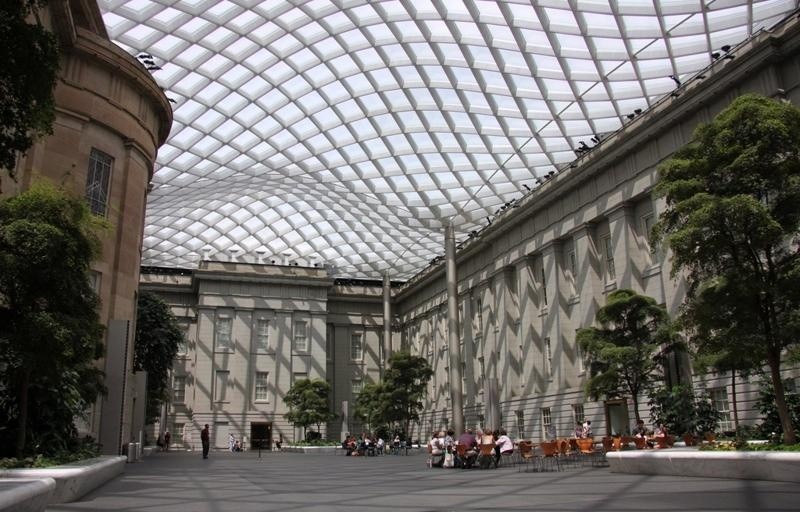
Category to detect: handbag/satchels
[443,446,454,467]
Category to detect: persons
[575,417,593,439]
[235,440,241,451]
[632,419,654,448]
[343,431,412,457]
[157,436,163,448]
[201,424,210,459]
[165,431,170,449]
[429,427,515,468]
[652,421,667,448]
[275,440,283,450]
[229,433,234,452]
[636,429,647,448]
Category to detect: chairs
[428,431,715,473]
[342,443,412,456]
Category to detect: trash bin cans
[127,443,136,462]
[135,442,142,460]
